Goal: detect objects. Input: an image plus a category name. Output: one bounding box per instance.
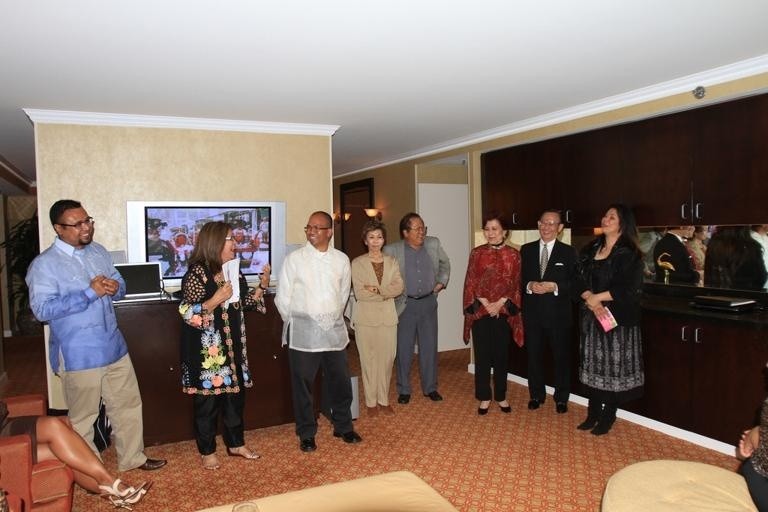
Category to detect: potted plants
[0,212,44,338]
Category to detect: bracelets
[259,283,266,290]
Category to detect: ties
[540,244,548,280]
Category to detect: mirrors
[340,178,375,260]
[637,225,768,290]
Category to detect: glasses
[226,236,235,241]
[60,217,93,229]
[410,227,427,233]
[304,225,328,231]
[538,220,560,226]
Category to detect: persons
[734,395,768,512]
[342,220,405,418]
[577,203,647,435]
[177,220,270,470]
[273,211,363,452]
[0,400,153,511]
[463,209,524,416]
[635,225,768,289]
[519,207,579,414]
[382,213,450,404]
[25,199,167,472]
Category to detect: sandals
[202,452,221,469]
[227,445,260,459]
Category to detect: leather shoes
[138,459,167,470]
[300,437,317,451]
[478,400,491,415]
[556,401,568,413]
[528,399,545,409]
[333,431,362,443]
[498,400,511,413]
[367,392,441,416]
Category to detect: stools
[600,460,762,512]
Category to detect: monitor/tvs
[126,199,287,300]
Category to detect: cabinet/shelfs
[480,137,566,230]
[506,307,768,459]
[564,123,624,227]
[626,93,767,225]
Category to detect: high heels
[577,407,602,429]
[591,410,617,435]
[99,479,147,507]
[110,481,153,511]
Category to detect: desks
[191,470,461,512]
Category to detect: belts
[407,291,434,300]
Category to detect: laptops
[112,261,167,303]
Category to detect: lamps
[342,213,352,224]
[333,212,341,224]
[364,208,382,226]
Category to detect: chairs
[0,395,75,512]
